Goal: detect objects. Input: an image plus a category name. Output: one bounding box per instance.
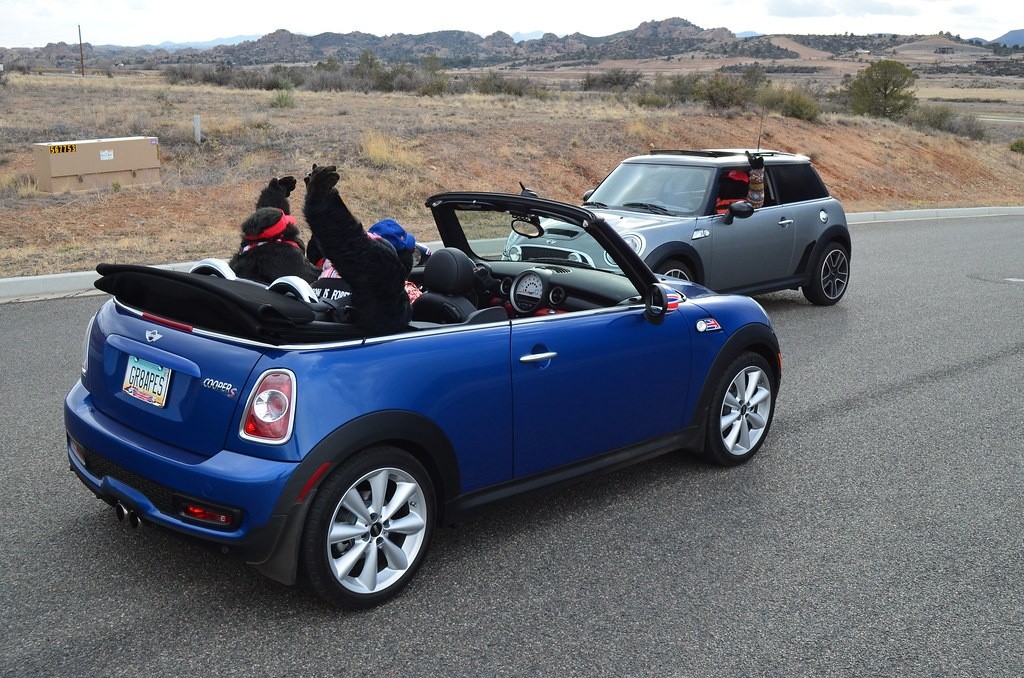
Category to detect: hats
[242,208,297,238]
[369,218,416,252]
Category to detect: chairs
[720,177,748,199]
[411,247,478,324]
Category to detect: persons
[315,216,423,304]
[716,150,765,215]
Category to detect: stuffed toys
[230,174,322,285]
[304,164,412,326]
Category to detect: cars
[64,179,782,612]
[499,148,852,305]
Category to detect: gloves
[745,150,764,170]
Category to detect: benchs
[187,257,336,322]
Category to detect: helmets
[729,169,749,183]
